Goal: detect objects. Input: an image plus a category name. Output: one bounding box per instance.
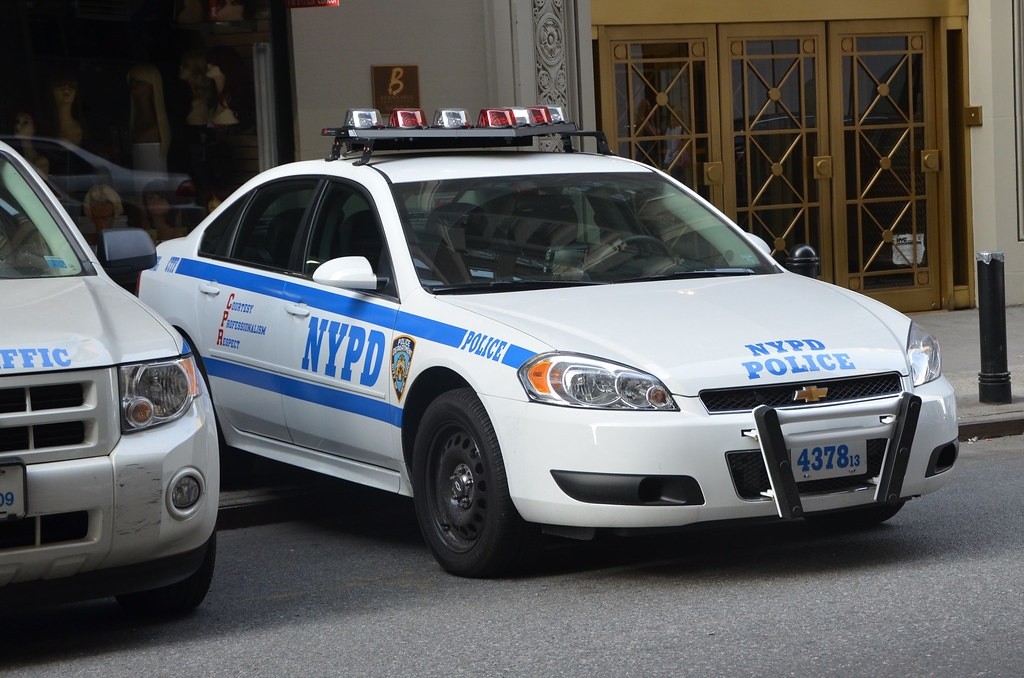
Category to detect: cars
[137,106,954,581]
[0,139,220,626]
[1,136,208,230]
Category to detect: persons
[0,56,247,273]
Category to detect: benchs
[267,205,390,278]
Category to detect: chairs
[377,226,467,295]
[509,215,552,270]
